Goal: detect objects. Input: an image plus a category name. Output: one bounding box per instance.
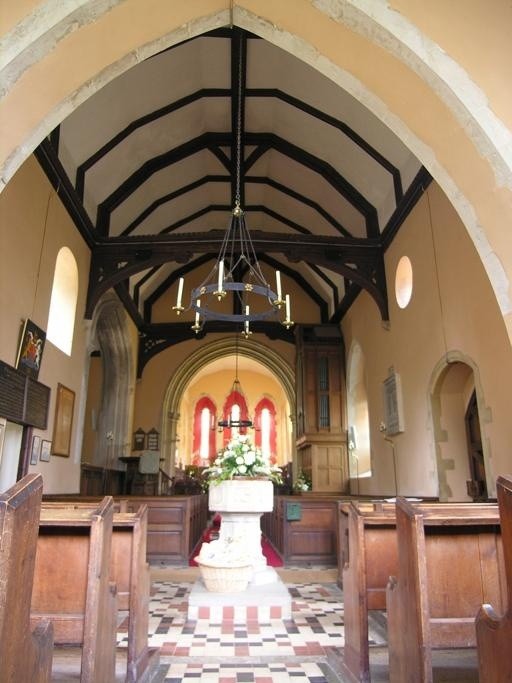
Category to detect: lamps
[173,38,299,339]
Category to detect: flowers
[202,433,286,485]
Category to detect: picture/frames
[39,439,53,463]
[382,372,404,436]
[30,435,42,465]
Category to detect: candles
[227,413,230,425]
[211,415,214,427]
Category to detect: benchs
[270,476,511,669]
[0,474,208,681]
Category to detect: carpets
[191,512,285,566]
